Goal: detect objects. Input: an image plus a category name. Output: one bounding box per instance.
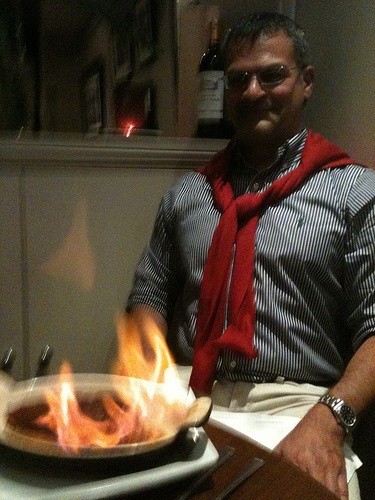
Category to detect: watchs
[318,394,356,433]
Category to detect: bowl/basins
[0,375,211,458]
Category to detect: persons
[125,13,375,500]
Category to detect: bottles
[195,16,229,139]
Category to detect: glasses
[218,65,300,91]
